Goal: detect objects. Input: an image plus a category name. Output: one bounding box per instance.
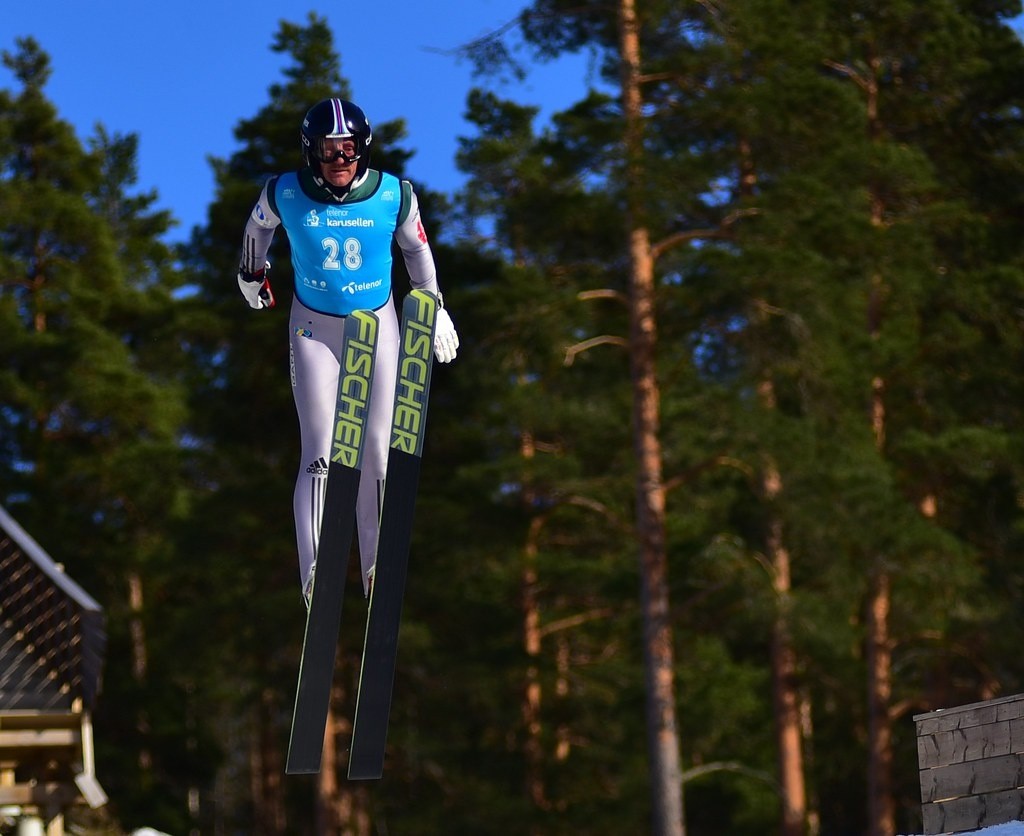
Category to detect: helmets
[301,97,372,201]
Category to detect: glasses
[309,135,364,163]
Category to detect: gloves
[434,291,460,363]
[238,261,271,309]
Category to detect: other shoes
[302,560,315,608]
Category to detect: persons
[238,98,460,607]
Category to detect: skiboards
[282,287,441,781]
